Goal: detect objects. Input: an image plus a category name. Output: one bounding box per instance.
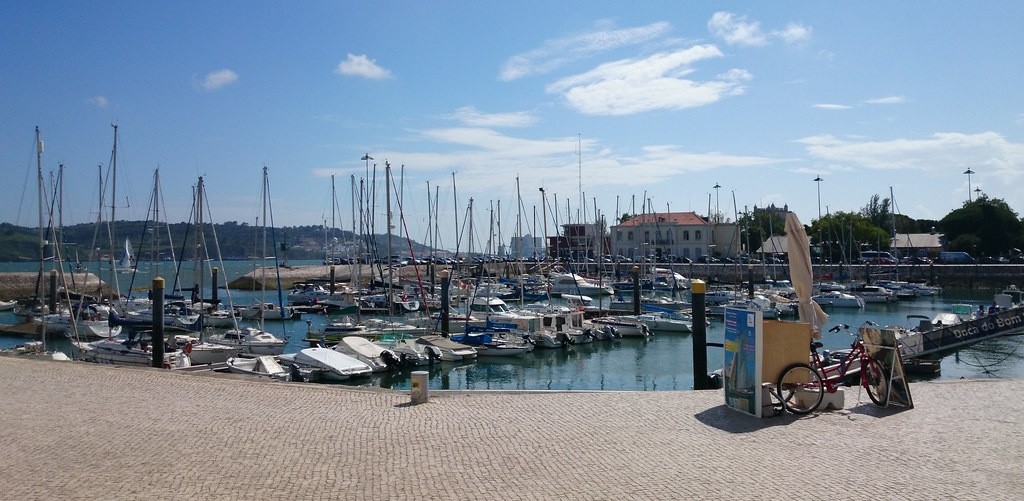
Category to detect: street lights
[963,167,974,202]
[974,186,982,200]
[813,175,825,221]
[712,181,722,224]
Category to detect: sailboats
[1,121,945,376]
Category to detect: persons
[988,301,1000,314]
[976,304,985,320]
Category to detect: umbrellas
[783,210,830,362]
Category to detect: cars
[996,257,1010,264]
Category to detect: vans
[859,250,900,264]
[940,252,976,265]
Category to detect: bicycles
[777,319,889,416]
